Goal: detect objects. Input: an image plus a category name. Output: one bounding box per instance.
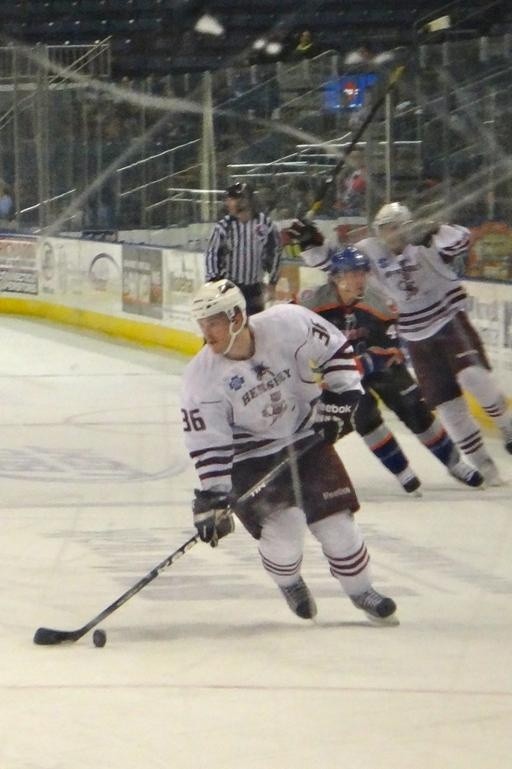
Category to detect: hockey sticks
[34,429,334,646]
[306,13,453,223]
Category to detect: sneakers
[477,455,499,483]
[447,459,486,488]
[505,439,512,455]
[278,577,318,619]
[347,585,398,620]
[395,464,421,494]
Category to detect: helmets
[191,278,250,326]
[371,201,414,230]
[223,182,253,198]
[329,244,371,273]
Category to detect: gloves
[279,218,324,260]
[354,350,381,380]
[400,222,440,248]
[193,487,236,549]
[311,390,362,446]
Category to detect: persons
[284,245,485,496]
[341,38,372,67]
[290,28,316,59]
[282,201,511,481]
[176,275,396,622]
[329,150,367,218]
[201,179,283,346]
[410,154,474,214]
